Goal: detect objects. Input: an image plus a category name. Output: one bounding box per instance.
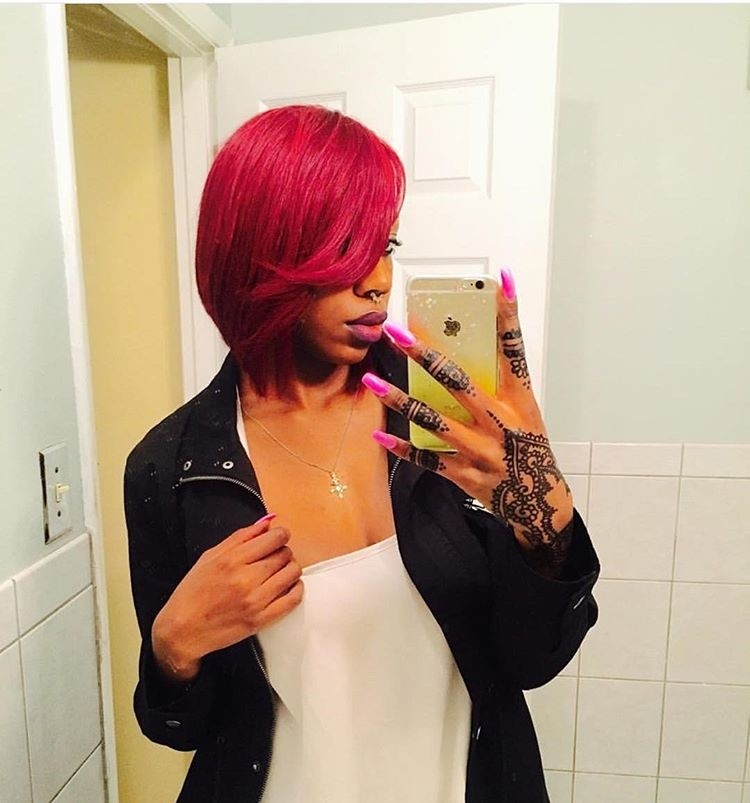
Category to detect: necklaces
[240,362,364,502]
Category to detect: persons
[123,103,602,803]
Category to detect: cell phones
[404,274,500,452]
